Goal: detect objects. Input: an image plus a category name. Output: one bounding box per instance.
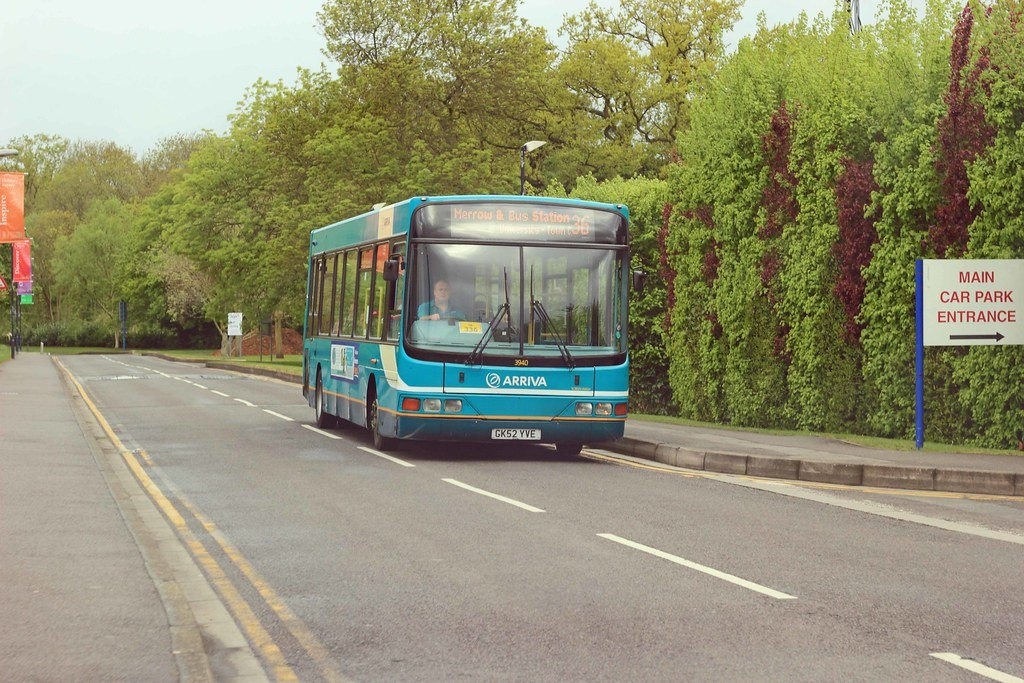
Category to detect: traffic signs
[917,258,1024,347]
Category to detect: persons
[343,302,353,336]
[418,280,467,321]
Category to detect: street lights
[519,139,548,194]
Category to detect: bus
[298,194,649,450]
